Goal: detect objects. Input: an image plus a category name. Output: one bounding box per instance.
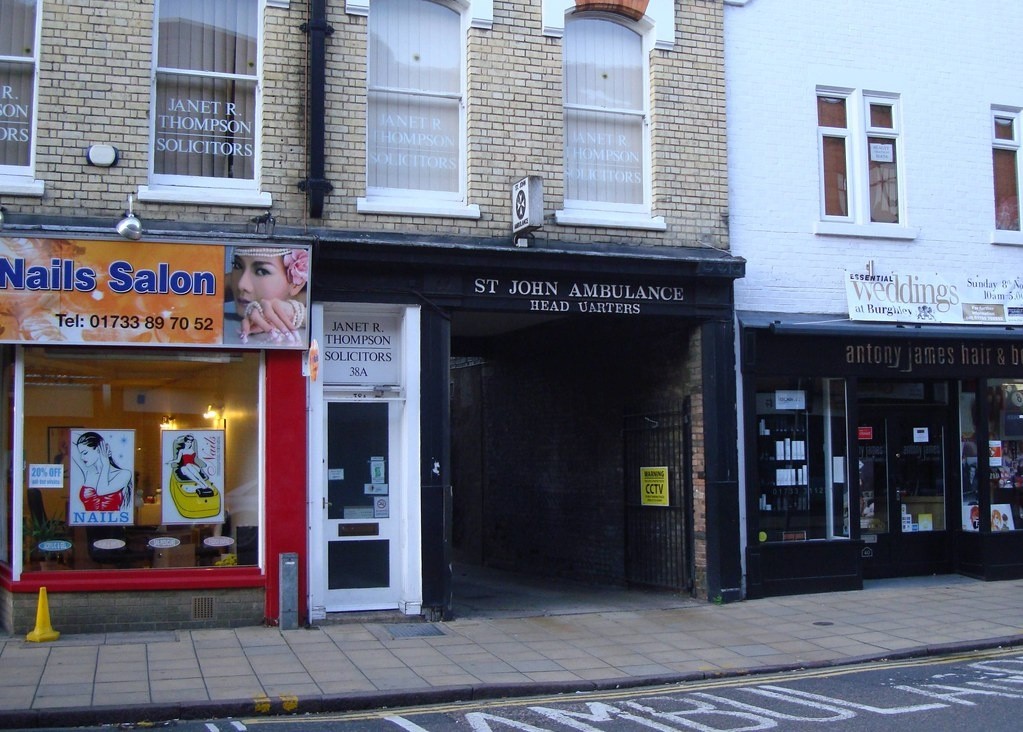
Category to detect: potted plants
[24,511,74,571]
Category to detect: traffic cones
[25,586,60,643]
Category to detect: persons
[965,465,978,500]
[223,248,308,344]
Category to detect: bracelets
[290,299,304,328]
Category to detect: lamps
[116,192,142,240]
[203,403,225,418]
[160,415,175,428]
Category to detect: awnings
[736,310,1023,339]
[0,212,747,277]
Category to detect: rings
[243,300,263,317]
